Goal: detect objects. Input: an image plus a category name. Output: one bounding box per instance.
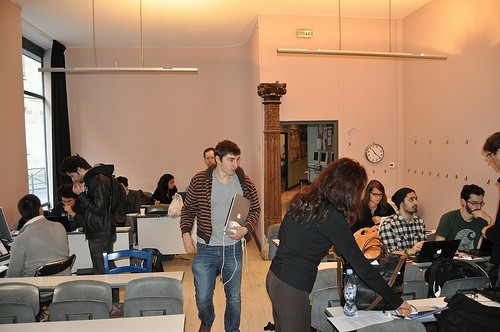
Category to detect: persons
[435,184,492,258]
[351,180,397,234]
[204,148,216,167]
[379,188,426,285]
[51,174,141,232]
[4,195,70,307]
[180,140,262,332]
[62,152,120,304]
[150,174,178,205]
[482,132,500,305]
[266,158,412,332]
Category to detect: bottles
[343,269,358,316]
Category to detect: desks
[0,271,185,292]
[0,313,187,332]
[272,239,490,271]
[125,211,198,255]
[66,227,132,276]
[325,292,500,332]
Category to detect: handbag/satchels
[168,192,184,218]
[353,225,381,259]
[433,291,500,332]
[340,236,406,311]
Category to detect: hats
[391,188,416,209]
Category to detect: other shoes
[198,316,215,332]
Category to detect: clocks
[365,143,384,163]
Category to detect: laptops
[409,239,462,263]
[44,216,73,232]
[224,193,251,226]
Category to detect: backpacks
[106,173,127,224]
[423,257,480,298]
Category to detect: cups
[140,208,146,215]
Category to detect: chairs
[0,188,500,332]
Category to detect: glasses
[468,201,486,207]
[371,192,383,197]
[486,153,492,159]
